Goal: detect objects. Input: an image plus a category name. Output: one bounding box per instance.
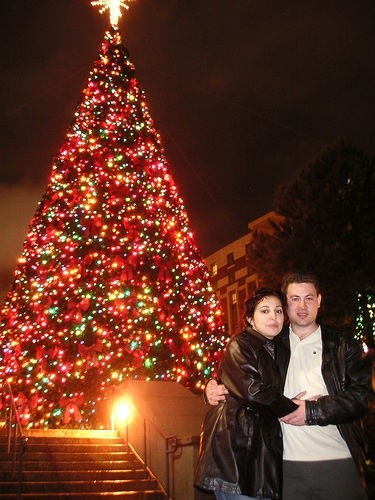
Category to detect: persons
[193,288,323,500]
[202,270,371,500]
